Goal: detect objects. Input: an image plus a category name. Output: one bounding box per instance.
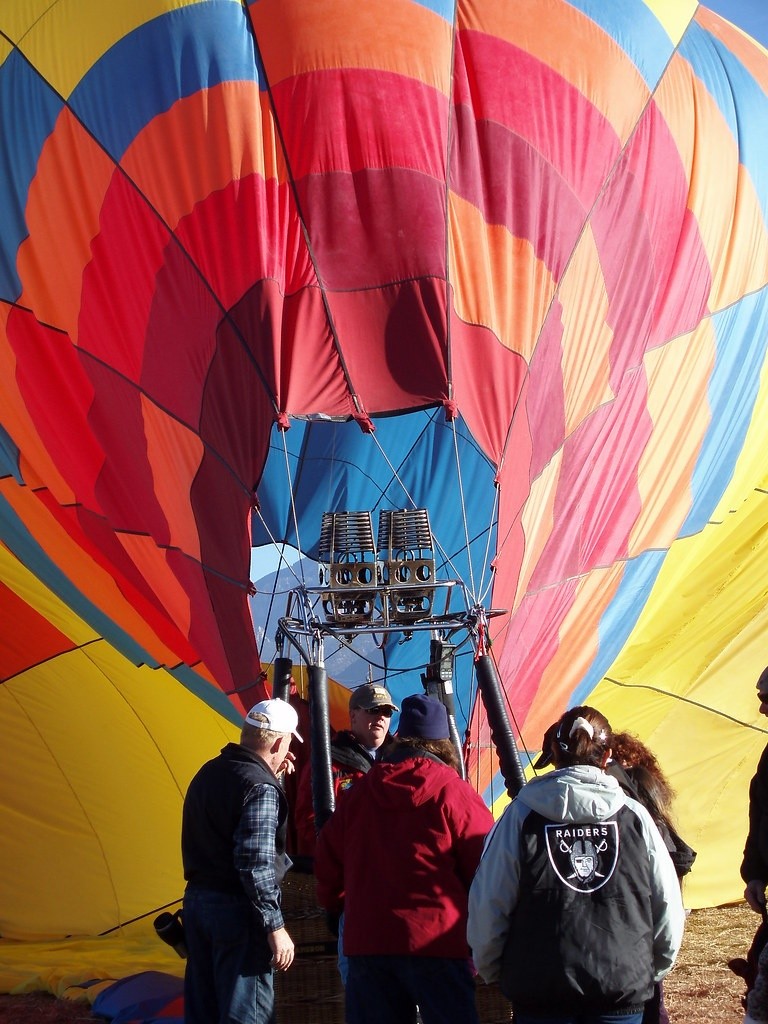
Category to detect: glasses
[757,693,768,703]
[364,706,394,718]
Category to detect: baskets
[272,870,347,1024]
[475,984,514,1024]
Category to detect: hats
[756,666,768,691]
[349,682,399,712]
[396,694,451,739]
[531,722,559,769]
[245,697,305,744]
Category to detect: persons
[296,684,495,1024]
[466,706,698,1024]
[181,697,303,1024]
[739,665,768,1024]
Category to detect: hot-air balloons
[2,2,768,1022]
